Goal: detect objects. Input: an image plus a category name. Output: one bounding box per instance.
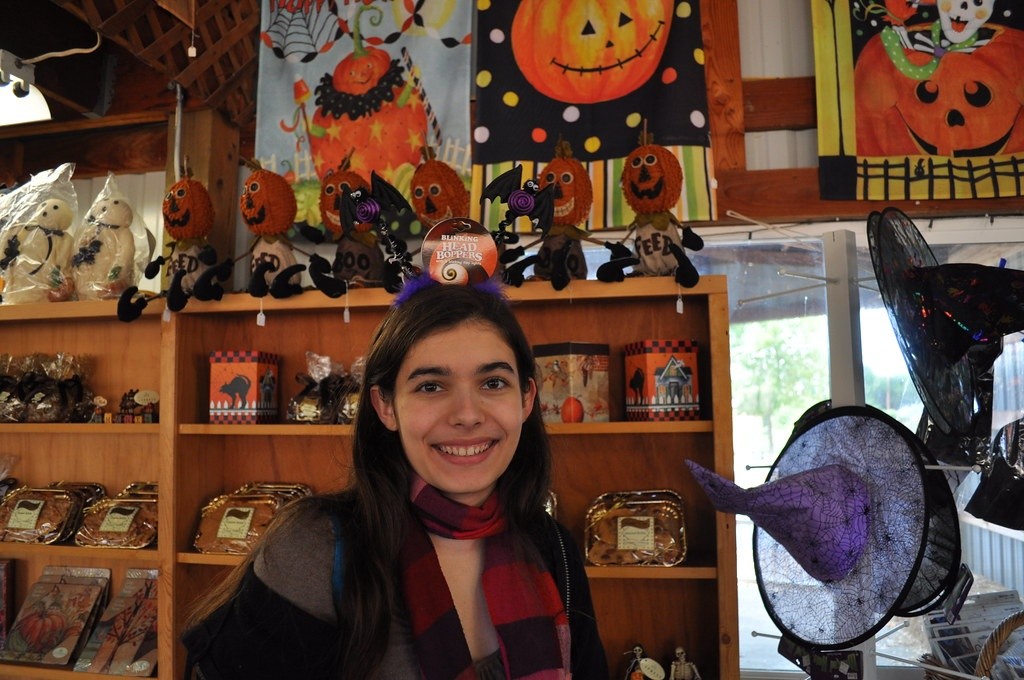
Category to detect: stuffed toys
[116,153,218,323]
[498,131,631,292]
[595,116,703,288]
[216,153,331,300]
[296,148,413,299]
[384,133,474,297]
[74,197,134,299]
[0,198,75,303]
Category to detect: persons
[178,274,608,680]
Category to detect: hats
[865,203,1024,436]
[683,399,968,651]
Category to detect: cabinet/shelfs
[0,275,740,680]
